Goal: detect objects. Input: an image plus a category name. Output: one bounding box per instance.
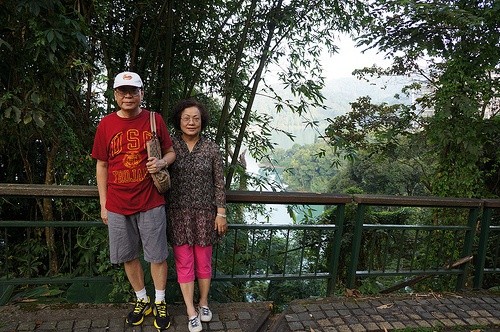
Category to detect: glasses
[114,87,141,95]
[180,114,203,122]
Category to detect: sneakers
[152,300,171,330]
[124,295,154,325]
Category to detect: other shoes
[197,305,213,322]
[187,312,203,332]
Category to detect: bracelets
[216,213,228,218]
[162,159,169,169]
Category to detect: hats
[112,71,144,89]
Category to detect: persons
[90,69,177,331]
[162,98,229,332]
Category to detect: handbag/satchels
[146,111,171,194]
